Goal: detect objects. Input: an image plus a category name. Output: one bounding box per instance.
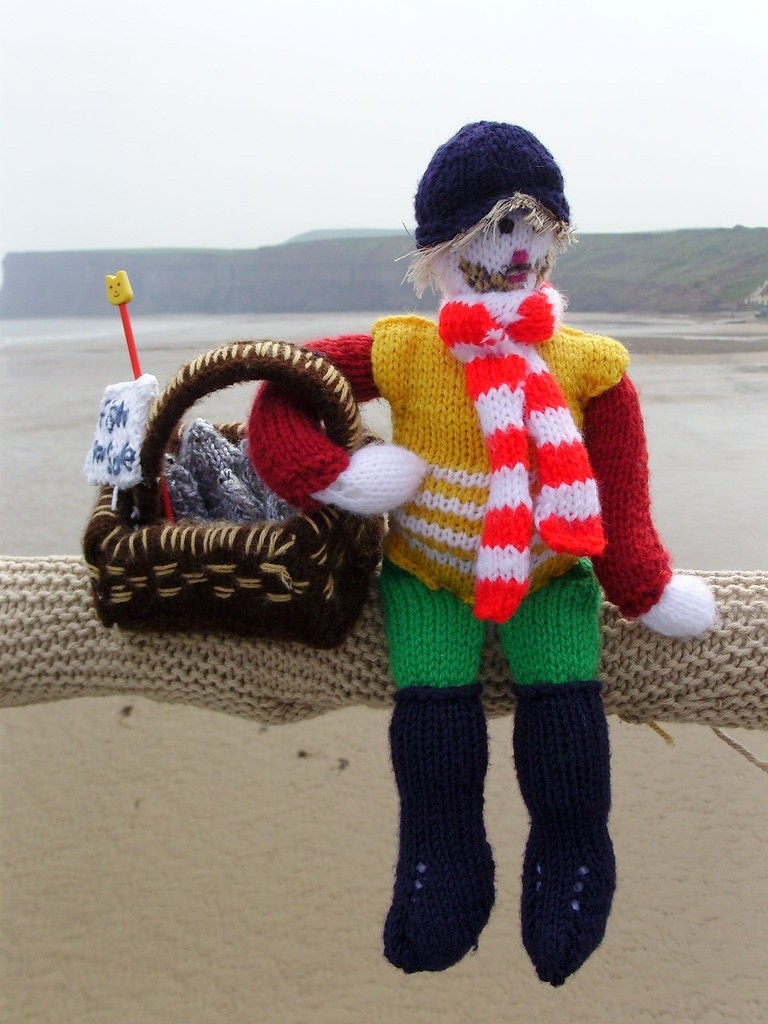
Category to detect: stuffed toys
[242,121,719,988]
